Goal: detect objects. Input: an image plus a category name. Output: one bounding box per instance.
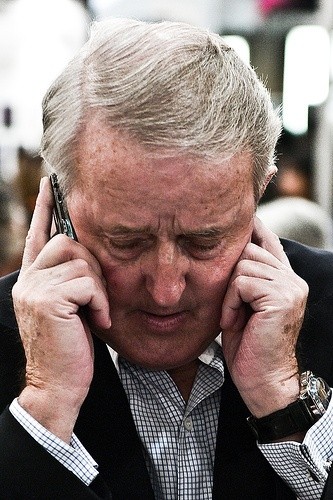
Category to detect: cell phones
[49,173,78,242]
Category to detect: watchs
[246,370,332,442]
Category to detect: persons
[0,23,333,500]
